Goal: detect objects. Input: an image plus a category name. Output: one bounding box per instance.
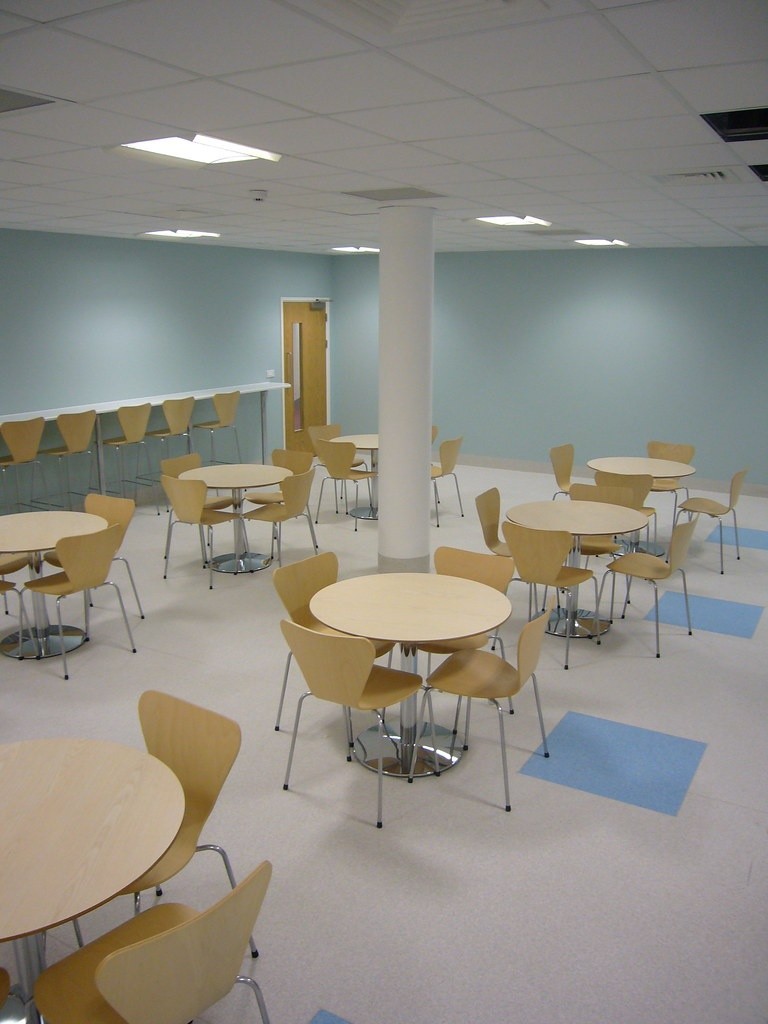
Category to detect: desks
[0,382,291,496]
[178,465,293,574]
[587,456,696,557]
[329,434,378,521]
[0,511,107,660]
[506,501,649,638]
[1,741,184,1024]
[309,573,511,777]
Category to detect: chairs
[0,392,749,830]
[27,858,274,1024]
[74,688,259,959]
